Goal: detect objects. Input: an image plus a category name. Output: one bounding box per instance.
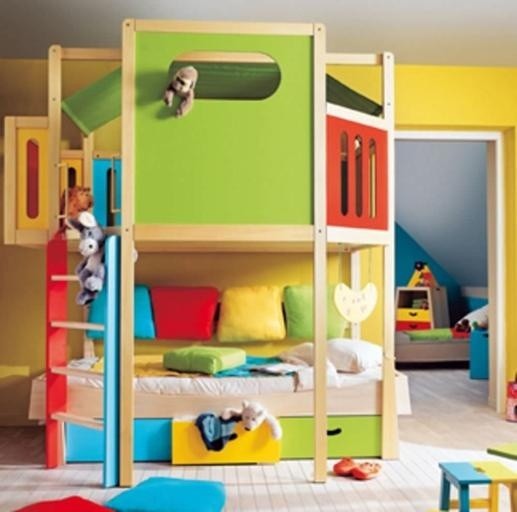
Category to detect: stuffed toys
[420,300,428,309]
[421,273,434,287]
[220,402,283,439]
[455,319,488,332]
[66,211,107,305]
[163,66,199,118]
[59,186,92,219]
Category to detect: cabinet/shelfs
[395,287,449,331]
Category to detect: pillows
[276,337,382,374]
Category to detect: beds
[393,326,470,370]
[25,350,413,462]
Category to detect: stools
[436,461,517,512]
